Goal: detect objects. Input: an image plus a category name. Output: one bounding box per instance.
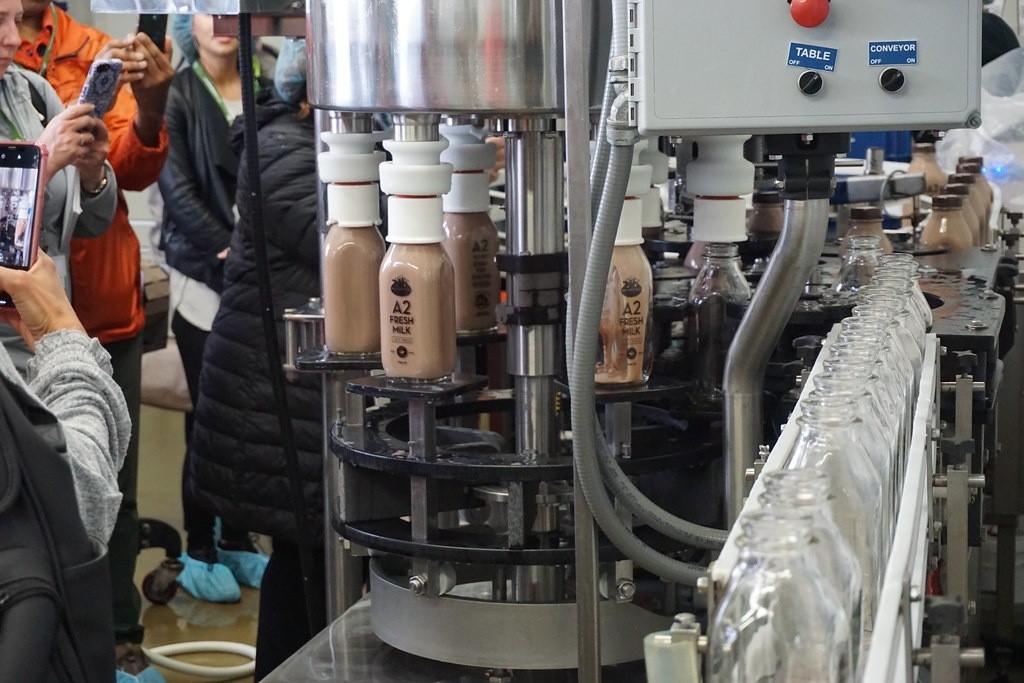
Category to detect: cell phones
[74,59,123,136]
[0,140,49,309]
[137,14,168,52]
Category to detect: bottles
[920,154,995,253]
[832,234,883,297]
[688,241,758,395]
[902,142,949,233]
[592,239,655,386]
[838,206,894,259]
[439,209,502,331]
[702,252,934,682]
[377,239,459,379]
[642,229,666,263]
[320,220,386,353]
[748,190,786,236]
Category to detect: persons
[930,13,1024,277]
[0,1,328,683]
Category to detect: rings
[80,133,84,145]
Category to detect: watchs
[90,178,107,194]
